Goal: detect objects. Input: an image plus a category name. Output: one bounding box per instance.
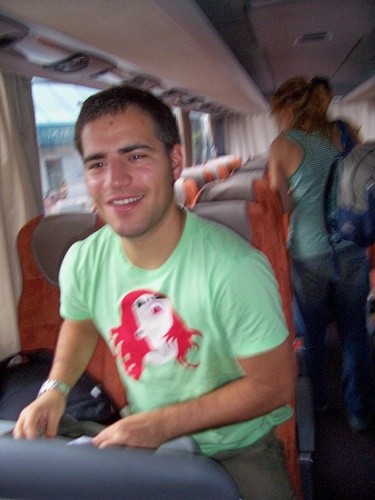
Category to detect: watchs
[37,378,70,399]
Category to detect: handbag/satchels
[1,348,111,425]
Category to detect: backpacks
[325,120,375,249]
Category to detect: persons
[268,75,374,433]
[12,86,296,500]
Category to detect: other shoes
[349,415,367,432]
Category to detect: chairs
[0,154,299,500]
